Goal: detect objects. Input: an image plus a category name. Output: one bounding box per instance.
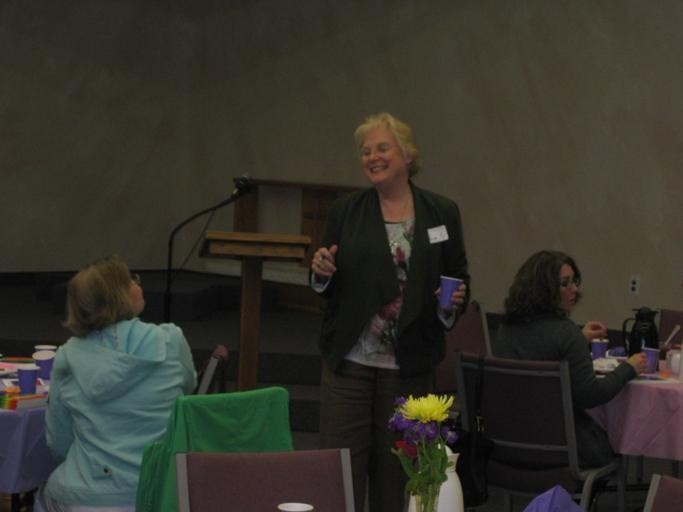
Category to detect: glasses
[132,273,143,285]
[563,276,580,288]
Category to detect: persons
[34,255,198,512]
[305,114,471,511]
[492,249,648,469]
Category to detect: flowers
[389,394,456,512]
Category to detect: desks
[586,346,682,488]
[2,351,80,512]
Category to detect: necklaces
[377,188,410,253]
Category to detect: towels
[523,483,584,512]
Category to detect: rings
[320,255,325,261]
[323,267,328,272]
[316,262,320,267]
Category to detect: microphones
[229,171,249,199]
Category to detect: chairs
[183,341,223,396]
[143,389,291,511]
[463,353,626,512]
[176,445,354,512]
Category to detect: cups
[32,350,56,380]
[641,347,660,374]
[34,345,57,353]
[17,367,40,396]
[592,339,609,360]
[438,276,463,310]
